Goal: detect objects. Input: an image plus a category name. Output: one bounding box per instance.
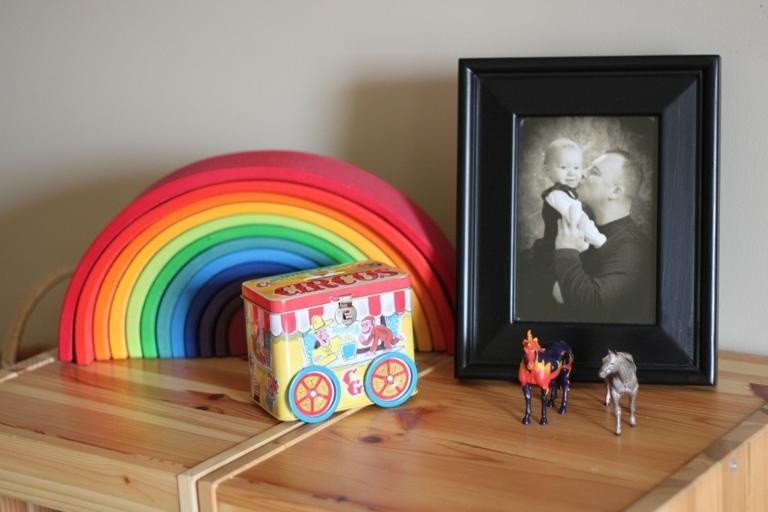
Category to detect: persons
[518,137,656,323]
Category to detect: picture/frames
[454,52,719,386]
